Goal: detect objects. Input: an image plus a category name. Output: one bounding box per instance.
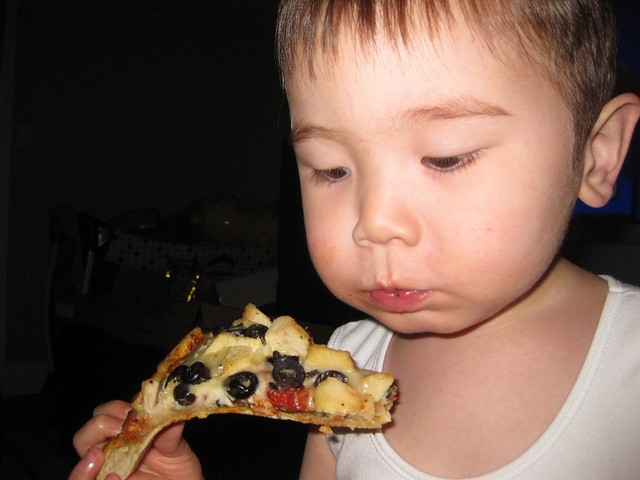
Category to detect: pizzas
[95,303,397,476]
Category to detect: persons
[69,1,640,479]
